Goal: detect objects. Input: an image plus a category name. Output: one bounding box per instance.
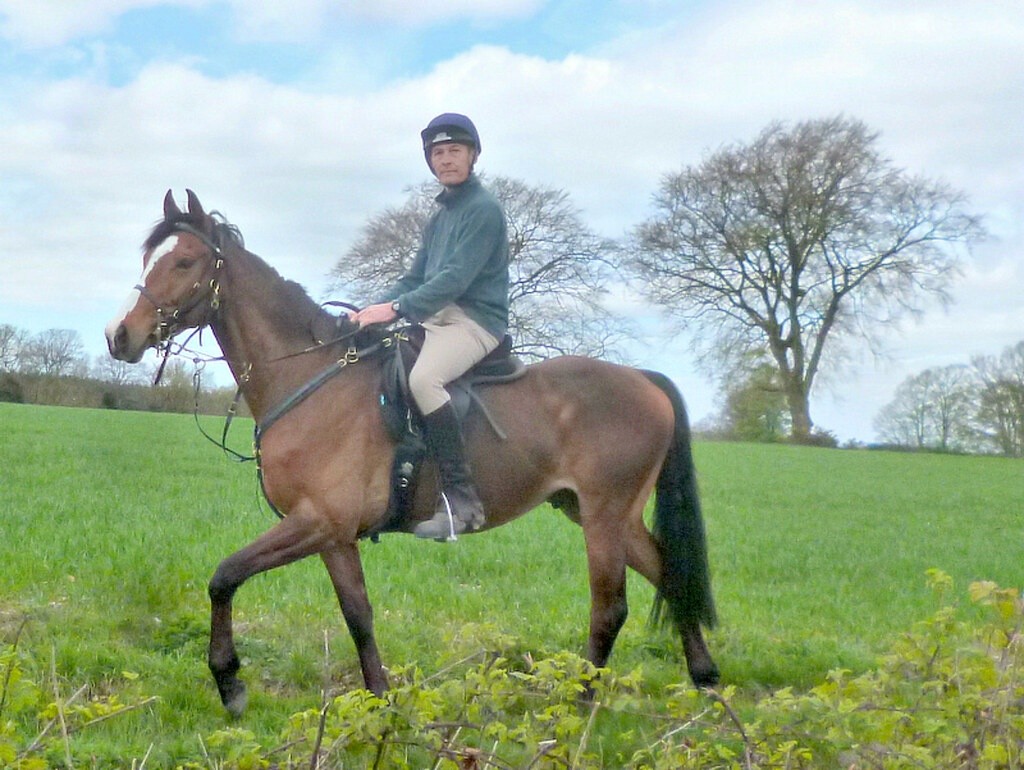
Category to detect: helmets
[421,112,481,177]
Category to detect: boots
[414,401,486,540]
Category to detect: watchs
[392,300,400,313]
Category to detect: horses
[102,186,724,724]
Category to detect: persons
[350,113,509,539]
